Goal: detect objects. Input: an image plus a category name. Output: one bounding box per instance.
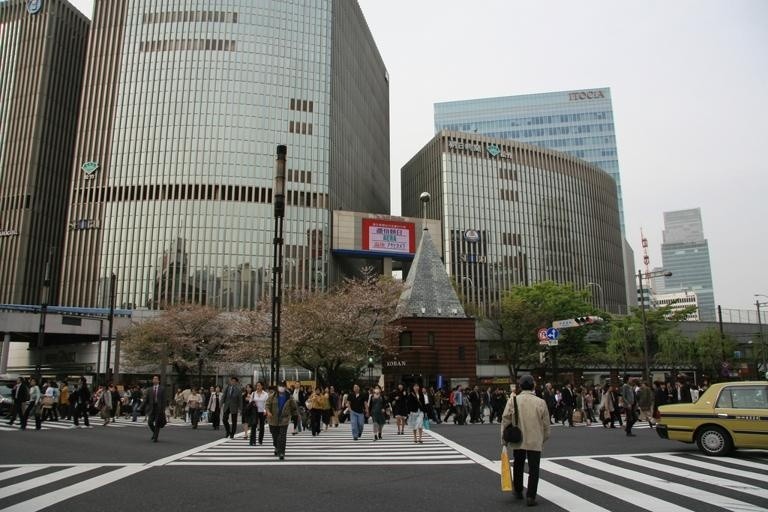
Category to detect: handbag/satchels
[423,417,432,432]
[303,399,313,411]
[41,395,53,406]
[499,450,511,492]
[502,424,525,445]
[158,413,168,430]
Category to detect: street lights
[754,294,768,300]
[462,275,474,303]
[638,268,673,387]
[588,282,605,312]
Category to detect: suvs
[61,382,94,398]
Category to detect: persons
[265,381,303,461]
[425,377,710,429]
[499,375,550,506]
[621,373,639,437]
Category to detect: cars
[654,378,767,457]
[116,385,128,415]
[0,382,15,414]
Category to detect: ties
[230,385,234,398]
[153,385,157,402]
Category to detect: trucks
[43,376,92,393]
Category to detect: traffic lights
[575,316,594,325]
[367,349,375,368]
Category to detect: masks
[276,386,286,393]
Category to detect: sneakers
[514,491,525,501]
[526,497,540,508]
